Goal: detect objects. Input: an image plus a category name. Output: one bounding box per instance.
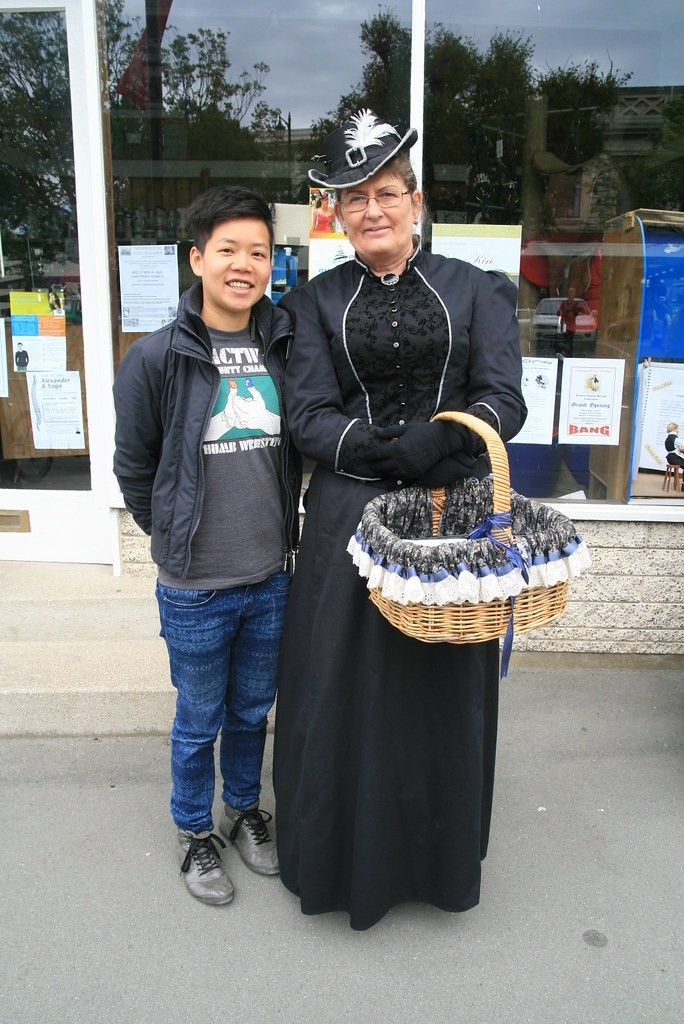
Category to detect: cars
[516,290,599,353]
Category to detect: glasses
[339,190,411,212]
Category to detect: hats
[307,107,418,188]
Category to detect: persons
[48,292,63,314]
[311,191,336,233]
[15,343,29,372]
[558,287,580,356]
[665,422,684,492]
[118,187,301,906]
[273,108,528,932]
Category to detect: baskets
[347,411,590,644]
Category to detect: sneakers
[177,825,234,905]
[219,800,280,876]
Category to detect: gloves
[422,451,475,489]
[364,420,465,482]
